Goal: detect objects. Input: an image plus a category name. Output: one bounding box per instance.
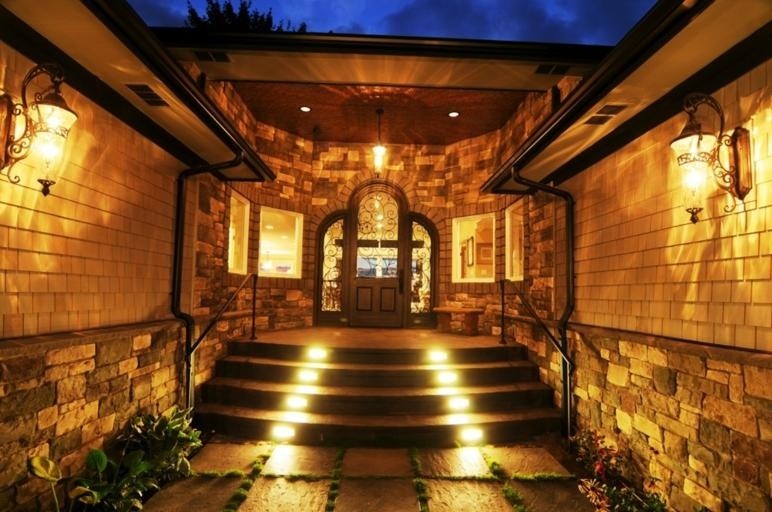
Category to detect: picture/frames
[476,243,494,264]
[466,236,474,267]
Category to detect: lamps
[0,62,81,195]
[371,106,387,174]
[669,91,753,224]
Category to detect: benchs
[433,306,484,336]
